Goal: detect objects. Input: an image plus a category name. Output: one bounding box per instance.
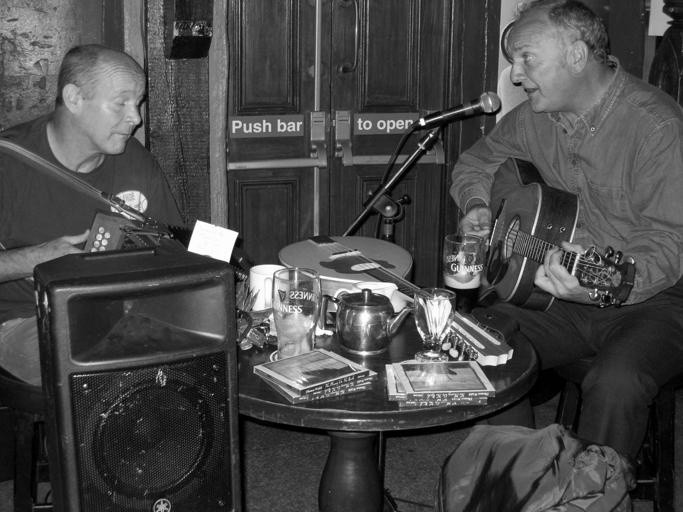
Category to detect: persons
[446,0,683,463]
[0,45,186,389]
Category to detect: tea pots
[320,288,412,358]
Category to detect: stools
[554,356,675,512]
[0,371,54,512]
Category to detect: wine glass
[410,287,457,366]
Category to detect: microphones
[413,91,502,128]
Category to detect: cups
[249,264,289,324]
[442,233,486,319]
[270,269,322,364]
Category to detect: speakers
[32,245,244,512]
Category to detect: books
[382,360,496,407]
[250,348,380,404]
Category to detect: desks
[238,316,537,511]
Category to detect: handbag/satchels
[434,422,636,511]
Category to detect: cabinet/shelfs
[225,0,501,289]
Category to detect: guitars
[471,157,636,310]
